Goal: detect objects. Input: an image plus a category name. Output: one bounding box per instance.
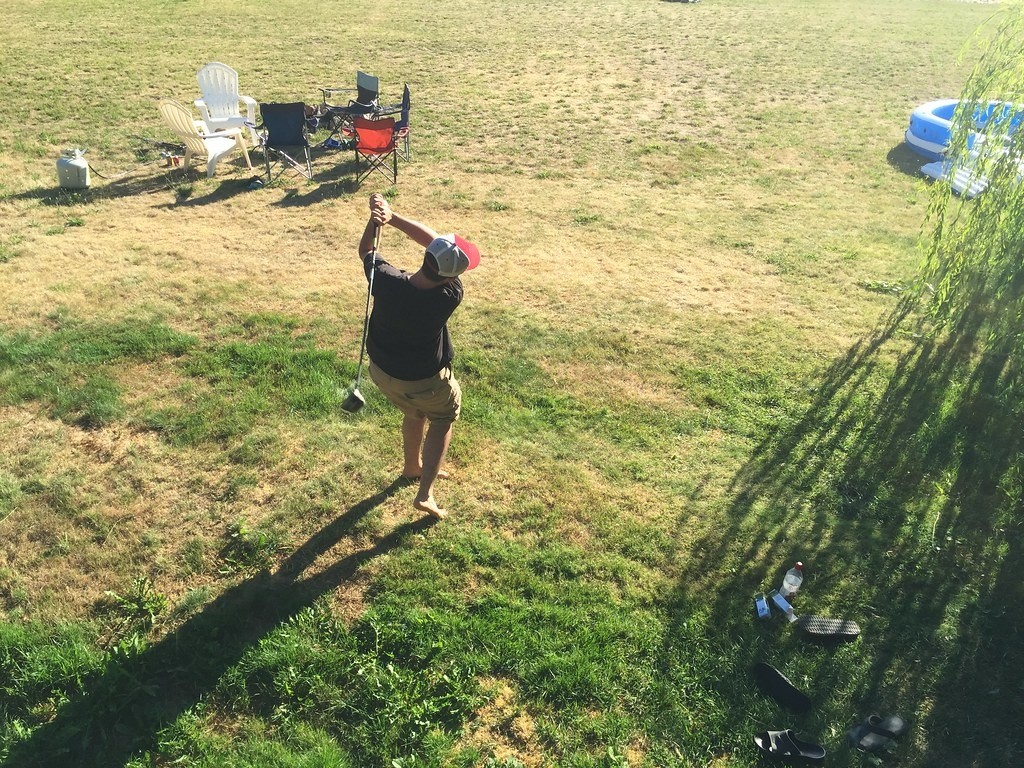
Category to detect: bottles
[779,562,803,603]
[166,151,172,166]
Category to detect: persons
[359,193,481,520]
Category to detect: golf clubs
[337,223,379,415]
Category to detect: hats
[424,233,481,278]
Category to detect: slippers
[847,713,906,750]
[753,728,827,761]
[799,614,861,636]
[755,660,811,713]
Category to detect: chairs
[370,84,410,162]
[244,102,313,186]
[158,99,253,178]
[342,117,398,184]
[194,63,259,152]
[318,70,380,149]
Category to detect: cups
[173,156,180,166]
